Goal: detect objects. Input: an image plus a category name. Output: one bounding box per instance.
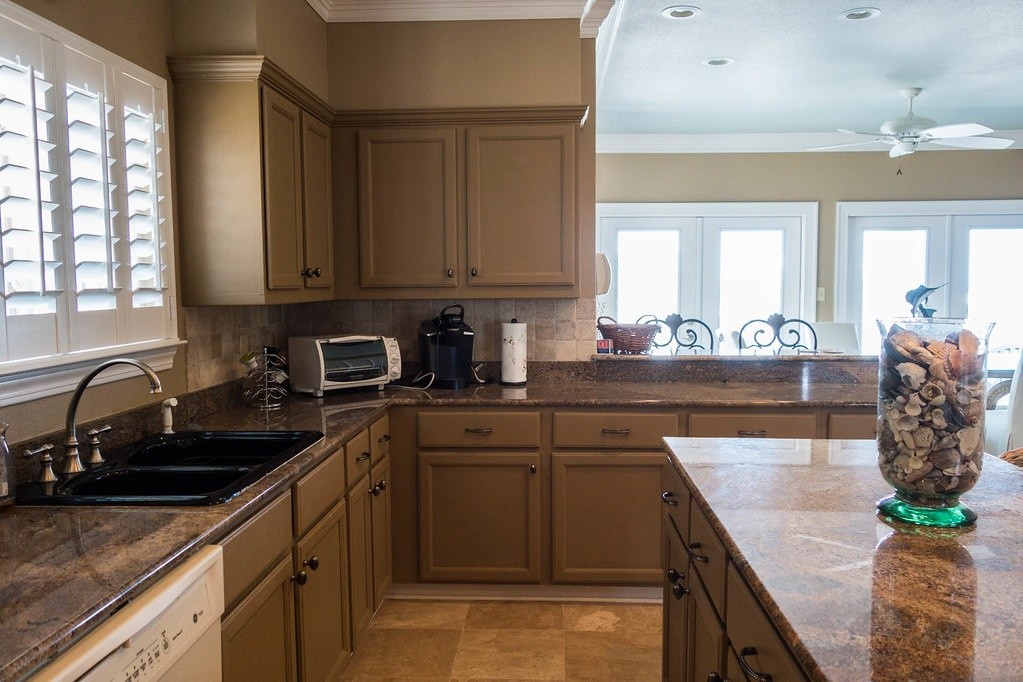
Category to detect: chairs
[714,328,748,350]
[735,313,820,350]
[806,318,861,350]
[635,313,716,349]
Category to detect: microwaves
[289,333,401,398]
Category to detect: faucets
[55,358,164,473]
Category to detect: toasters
[420,304,474,389]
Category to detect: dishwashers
[17,544,226,682]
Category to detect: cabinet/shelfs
[167,56,340,304]
[660,430,1022,680]
[340,415,409,654]
[392,403,544,583]
[691,411,820,443]
[549,405,683,590]
[215,446,357,681]
[349,107,592,301]
[829,411,891,443]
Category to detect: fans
[805,83,1016,160]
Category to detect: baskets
[597,321,661,353]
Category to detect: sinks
[103,431,324,467]
[18,467,277,508]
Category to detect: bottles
[874,315,996,526]
[500,319,528,386]
[0,421,17,507]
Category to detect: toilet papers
[501,322,527,382]
